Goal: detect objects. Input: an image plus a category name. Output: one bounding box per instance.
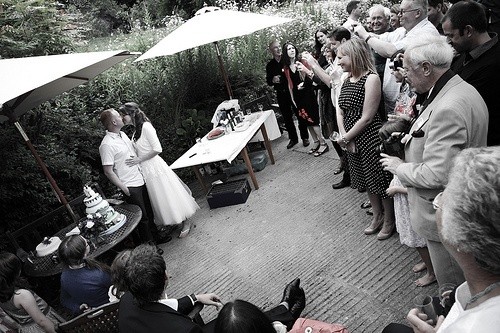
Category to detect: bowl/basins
[233,121,249,132]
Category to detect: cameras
[389,58,403,71]
[382,136,405,161]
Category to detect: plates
[207,130,225,140]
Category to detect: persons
[266,0,500,293]
[0,252,68,333]
[383,146,500,333]
[217,299,347,333]
[110,243,305,333]
[99,102,200,246]
[61,234,110,315]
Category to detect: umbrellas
[133,2,294,114]
[0,49,141,227]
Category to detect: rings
[132,162,133,164]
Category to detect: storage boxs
[206,178,252,210]
[224,150,268,177]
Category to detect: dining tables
[24,204,143,276]
[169,110,281,190]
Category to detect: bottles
[230,111,238,126]
[227,113,236,131]
[238,108,244,122]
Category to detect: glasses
[122,114,130,117]
[400,8,419,14]
[433,192,444,211]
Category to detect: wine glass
[258,105,263,111]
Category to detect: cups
[195,137,202,144]
[415,294,439,327]
[388,111,396,115]
[246,108,251,115]
[329,131,340,141]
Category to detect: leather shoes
[279,278,300,309]
[290,287,305,321]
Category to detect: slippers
[360,198,372,209]
[179,221,193,238]
[366,207,373,215]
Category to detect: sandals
[309,140,320,154]
[314,143,329,157]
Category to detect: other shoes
[287,137,298,149]
[303,139,310,147]
[156,236,172,245]
[332,180,350,189]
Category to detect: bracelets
[190,293,197,302]
[307,71,312,77]
[341,136,349,144]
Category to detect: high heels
[363,217,384,235]
[377,225,395,240]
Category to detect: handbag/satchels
[287,316,346,333]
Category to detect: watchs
[365,34,373,43]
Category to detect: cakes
[35,236,62,256]
[83,185,121,231]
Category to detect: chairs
[58,301,131,333]
